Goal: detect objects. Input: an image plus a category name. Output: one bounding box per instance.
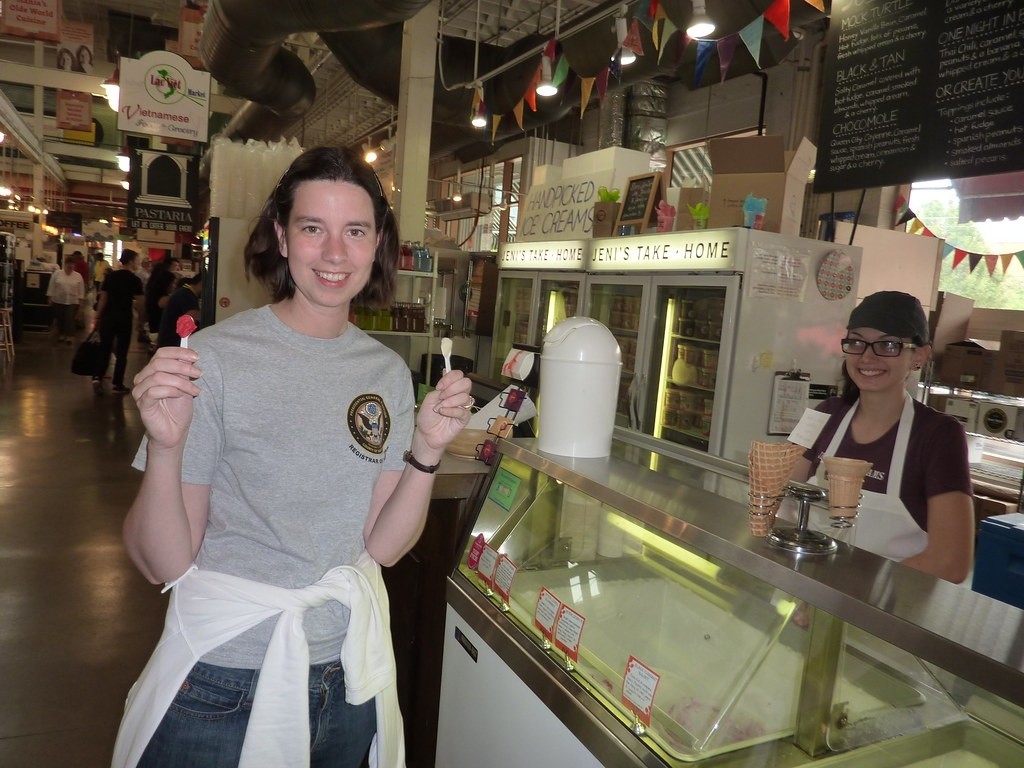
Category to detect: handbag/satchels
[71,329,102,376]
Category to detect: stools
[0,307,15,361]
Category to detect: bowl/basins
[446,429,513,462]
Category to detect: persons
[45,250,206,392]
[57,46,95,74]
[110,148,474,768]
[789,290,974,701]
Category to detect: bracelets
[403,449,441,473]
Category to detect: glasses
[841,338,919,358]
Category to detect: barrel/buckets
[420,353,474,387]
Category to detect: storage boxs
[552,147,651,239]
[592,201,621,239]
[707,134,817,240]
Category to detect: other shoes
[137,334,151,344]
[111,382,131,394]
[91,376,105,397]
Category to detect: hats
[847,290,929,346]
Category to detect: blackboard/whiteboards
[813,0,1024,192]
[615,170,668,225]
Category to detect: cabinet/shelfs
[347,248,439,384]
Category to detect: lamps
[116,146,130,173]
[535,49,558,97]
[471,81,488,129]
[380,127,397,152]
[685,0,715,38]
[611,4,637,64]
[99,51,126,112]
[361,137,377,163]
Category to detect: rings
[459,396,475,409]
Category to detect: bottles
[398,239,431,272]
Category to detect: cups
[210,139,302,219]
[597,520,621,559]
[664,388,713,436]
[349,304,424,332]
[744,210,766,230]
[677,343,718,389]
[678,299,721,341]
[657,215,674,233]
[514,286,640,371]
[692,218,708,229]
